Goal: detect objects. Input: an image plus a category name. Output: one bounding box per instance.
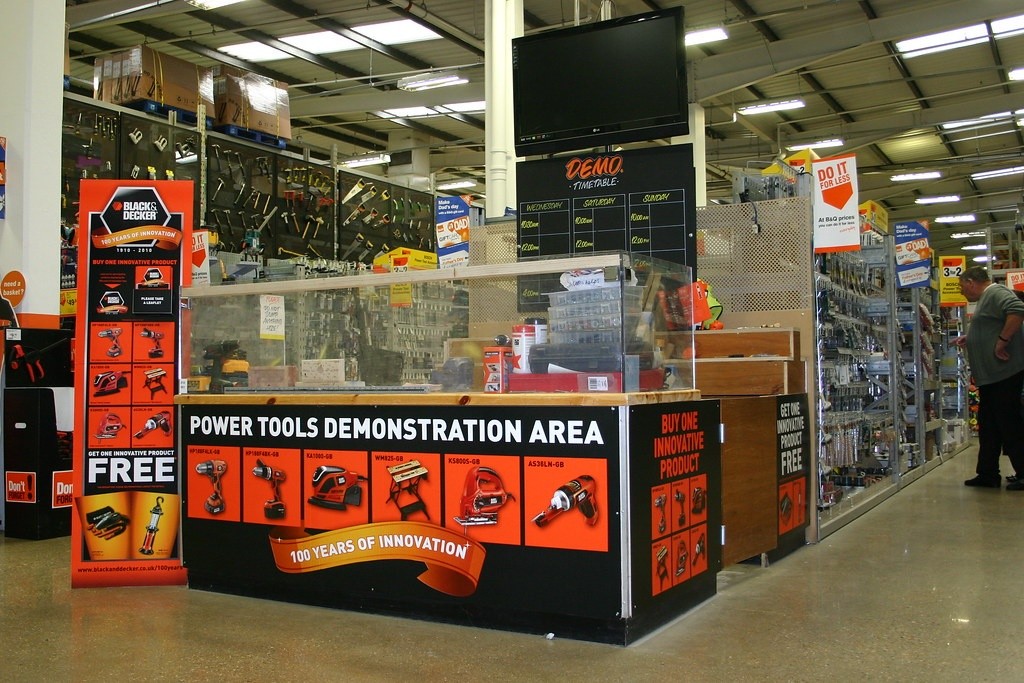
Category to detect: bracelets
[998,335,1009,342]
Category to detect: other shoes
[1007,473,1020,480]
[964,472,1001,488]
[1006,478,1024,491]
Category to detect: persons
[952,266,1024,491]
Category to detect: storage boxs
[94,44,215,119]
[208,63,292,143]
[482,285,665,394]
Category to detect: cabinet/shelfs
[733,158,971,544]
[446,326,807,395]
[986,226,1024,284]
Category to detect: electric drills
[693,533,705,565]
[195,458,287,519]
[654,490,686,532]
[533,475,599,527]
[203,338,242,393]
[98,327,171,438]
[308,464,368,511]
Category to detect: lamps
[685,21,728,47]
[341,150,391,168]
[784,136,844,152]
[436,177,477,190]
[736,99,805,115]
[397,70,469,92]
[882,15,1024,263]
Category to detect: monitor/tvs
[511,6,689,157]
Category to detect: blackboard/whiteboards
[515,143,696,312]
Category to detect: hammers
[277,212,324,259]
[212,176,271,214]
[211,144,246,179]
[211,208,272,252]
[284,165,336,210]
